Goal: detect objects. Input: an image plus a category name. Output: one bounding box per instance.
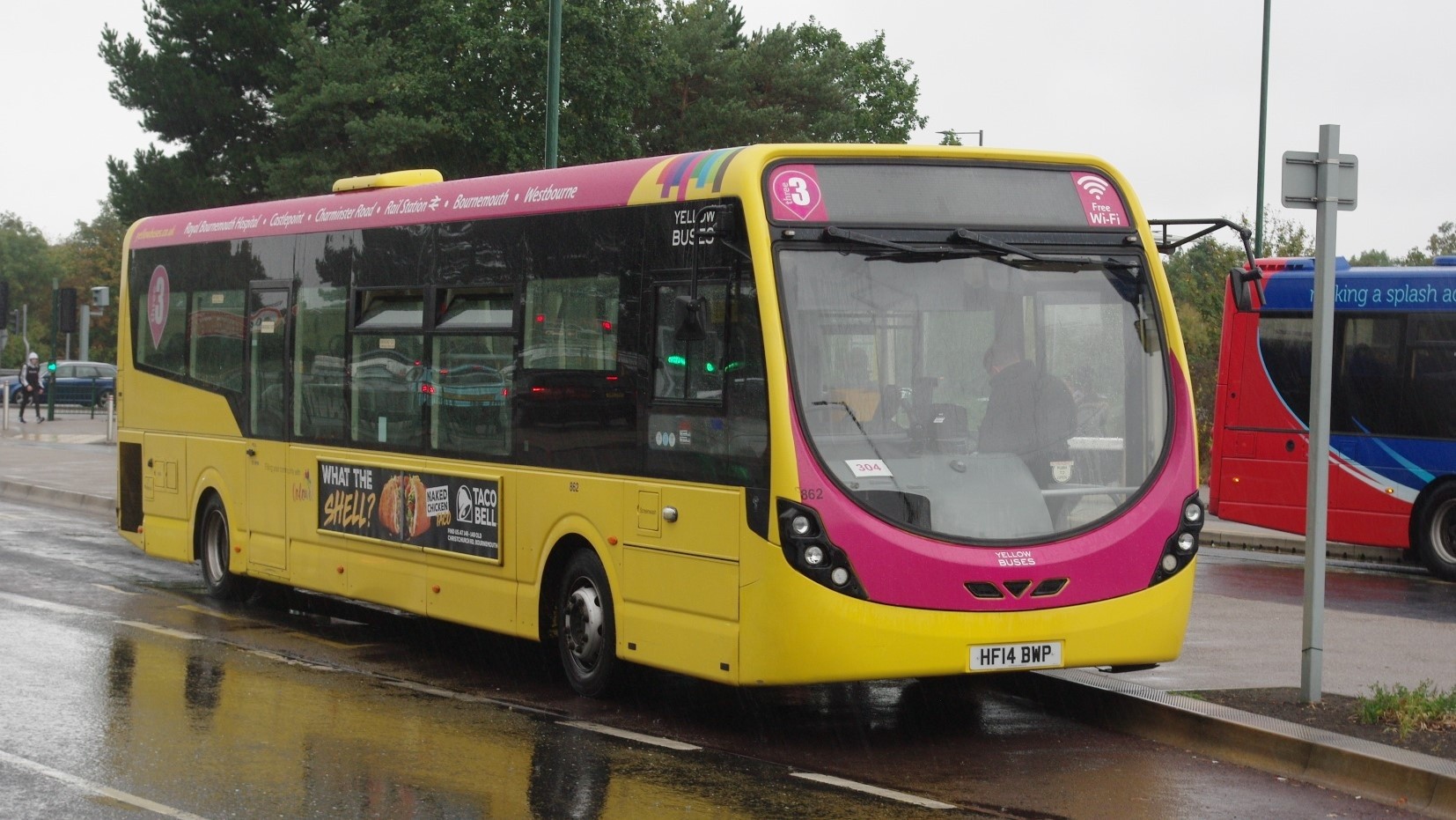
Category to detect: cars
[0,361,117,406]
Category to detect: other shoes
[19,416,26,424]
[37,415,45,424]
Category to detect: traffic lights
[47,359,56,374]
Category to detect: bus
[1206,254,1456,581]
[113,140,1266,700]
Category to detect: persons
[980,343,1076,520]
[18,352,44,423]
